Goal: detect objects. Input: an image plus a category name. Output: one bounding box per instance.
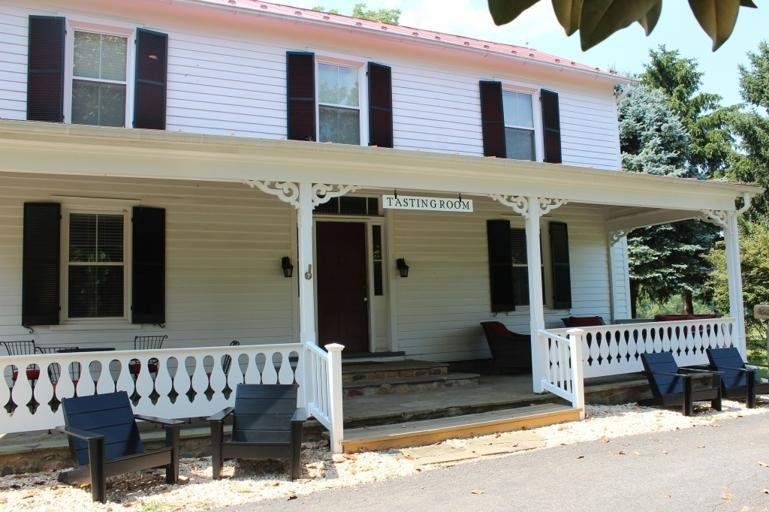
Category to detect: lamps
[282,257,293,277]
[397,258,408,277]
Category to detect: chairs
[641,346,769,417]
[56,382,308,503]
[480,315,607,372]
[3,333,241,435]
